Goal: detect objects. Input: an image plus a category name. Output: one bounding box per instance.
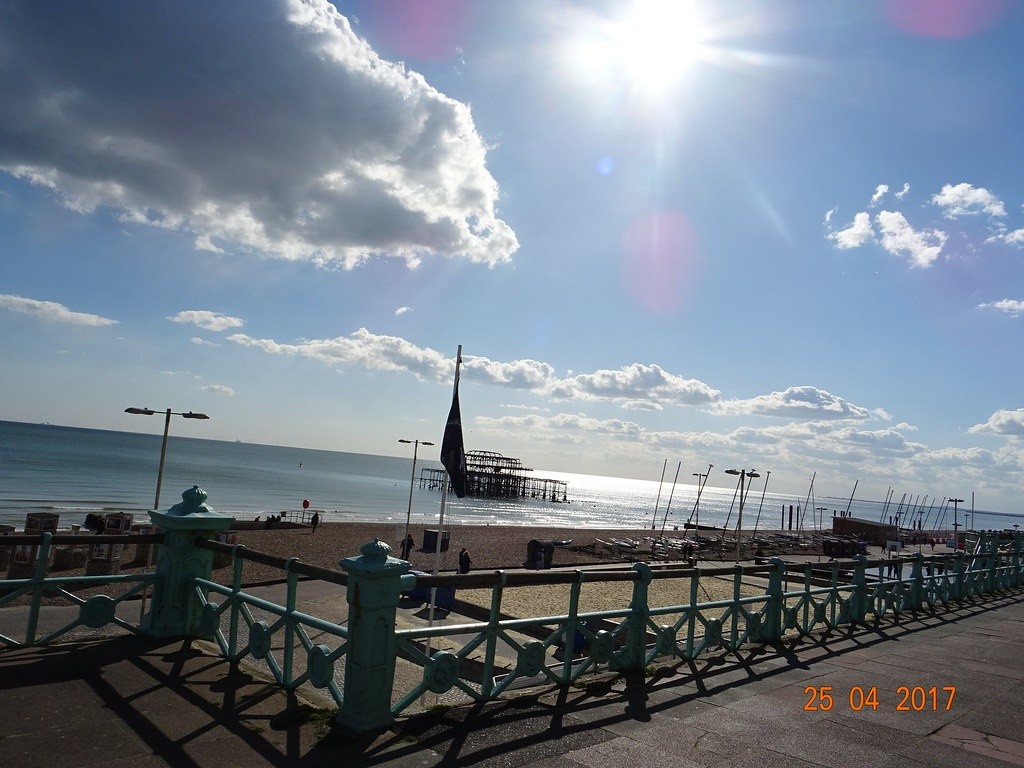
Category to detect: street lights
[948,498,964,554]
[693,473,707,541]
[951,523,962,550]
[725,469,760,564]
[896,511,904,528]
[123,407,211,570]
[398,438,434,560]
[963,514,970,530]
[917,510,925,530]
[816,507,827,531]
[1013,523,1021,531]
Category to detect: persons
[931,539,935,551]
[882,541,887,554]
[682,543,694,560]
[888,565,898,578]
[459,548,472,574]
[901,539,904,549]
[912,536,917,547]
[267,515,281,522]
[311,512,319,533]
[255,516,260,522]
[400,534,415,561]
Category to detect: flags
[440,384,468,498]
